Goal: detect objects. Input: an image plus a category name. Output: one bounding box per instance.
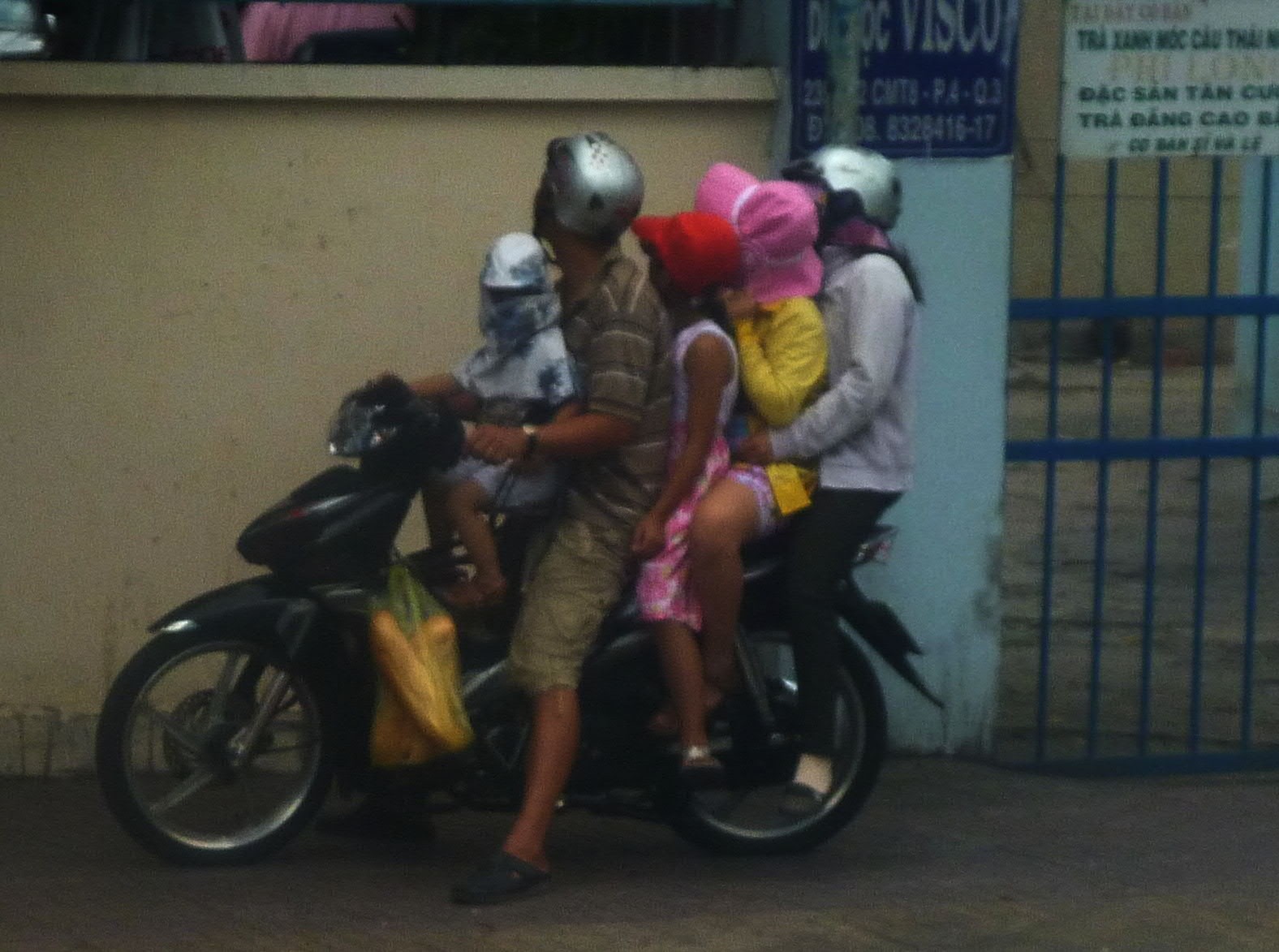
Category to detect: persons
[410,132,927,905]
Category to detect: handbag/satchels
[362,563,472,767]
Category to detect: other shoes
[436,574,511,614]
[645,700,682,739]
[681,745,718,771]
[786,746,845,818]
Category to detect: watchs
[522,424,537,453]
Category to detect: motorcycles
[96,372,950,869]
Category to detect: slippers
[451,851,546,904]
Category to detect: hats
[482,232,545,292]
[630,212,744,293]
[697,163,825,307]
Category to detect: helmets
[542,134,645,244]
[783,142,903,230]
